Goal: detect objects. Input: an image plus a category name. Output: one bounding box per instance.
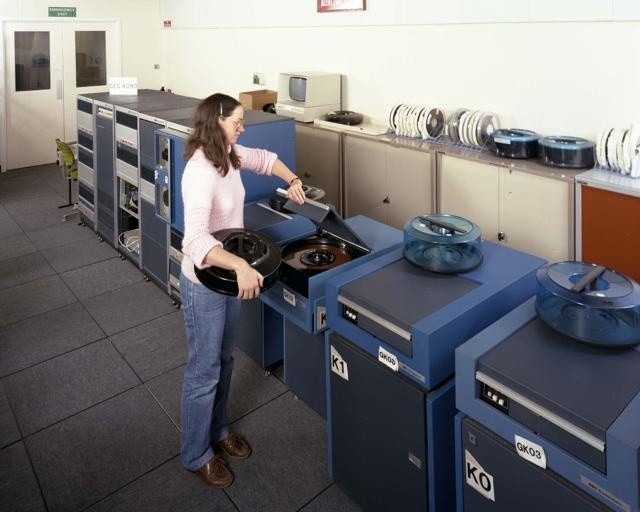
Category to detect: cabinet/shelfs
[575,171,640,285]
[343,135,432,232]
[297,124,341,218]
[453,294,640,512]
[76,91,296,302]
[233,295,326,421]
[436,154,575,265]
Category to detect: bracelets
[290,175,301,184]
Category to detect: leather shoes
[220,432,251,459]
[196,455,233,489]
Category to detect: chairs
[54,137,85,224]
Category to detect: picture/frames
[316,0,367,13]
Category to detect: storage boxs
[238,89,278,112]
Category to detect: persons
[180,93,307,488]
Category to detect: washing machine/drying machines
[456,260,640,512]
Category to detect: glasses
[224,117,244,127]
